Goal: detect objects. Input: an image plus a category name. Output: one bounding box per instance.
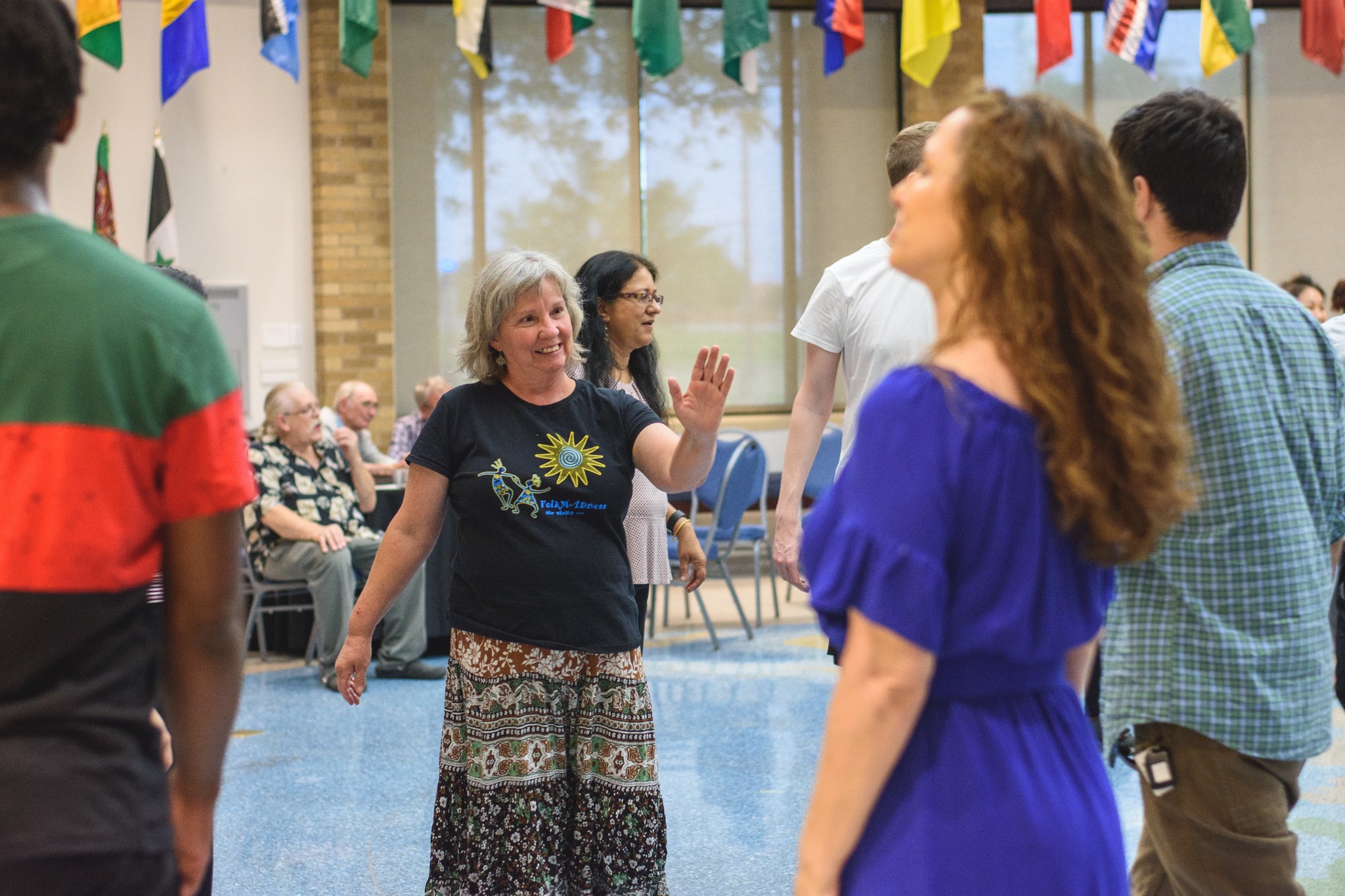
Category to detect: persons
[0,0,245,896]
[240,251,733,896]
[777,91,1345,896]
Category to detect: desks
[248,484,451,657]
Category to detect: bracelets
[666,510,686,535]
[672,519,690,541]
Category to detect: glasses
[283,405,321,416]
[617,293,663,306]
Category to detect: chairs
[237,518,371,667]
[643,424,845,653]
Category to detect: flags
[538,0,595,67]
[452,0,495,80]
[631,0,684,80]
[338,1,378,81]
[260,0,301,85]
[146,137,180,271]
[94,131,119,251]
[159,1,209,103]
[75,0,123,69]
[716,0,1345,99]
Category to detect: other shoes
[320,666,368,693]
[375,660,446,679]
